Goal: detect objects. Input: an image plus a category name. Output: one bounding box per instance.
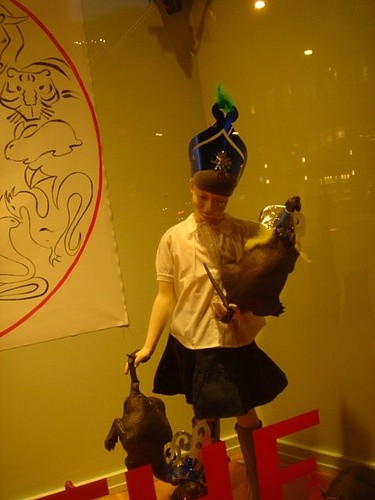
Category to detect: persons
[124,158,290,500]
